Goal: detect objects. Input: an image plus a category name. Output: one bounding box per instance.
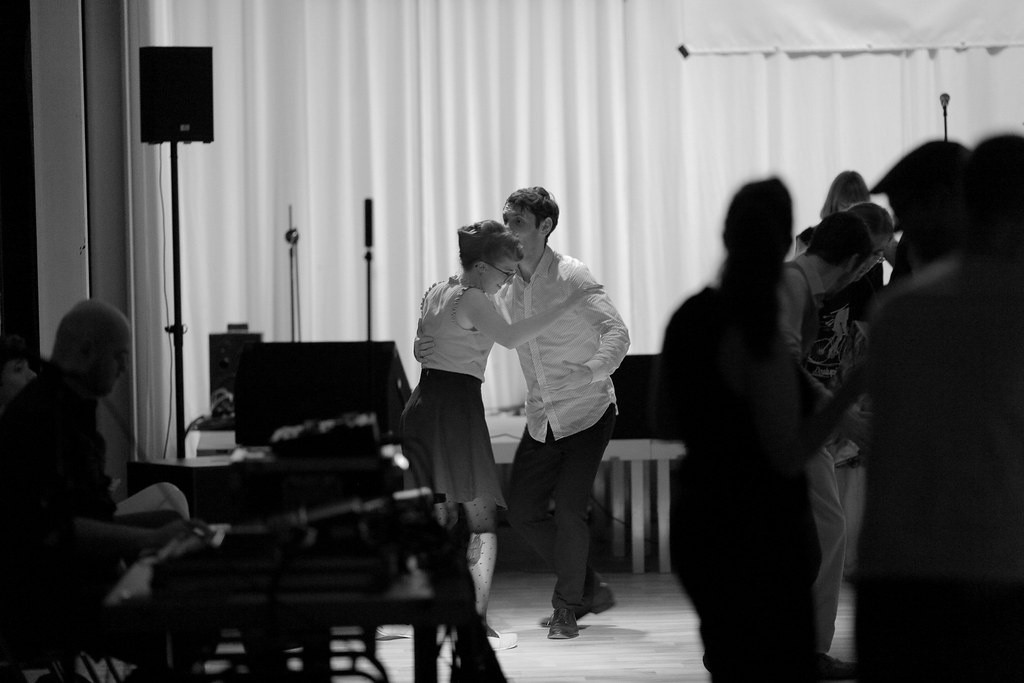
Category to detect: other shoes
[487,627,518,651]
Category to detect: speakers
[139,45,214,143]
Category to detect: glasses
[480,258,517,283]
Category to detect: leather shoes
[541,582,616,639]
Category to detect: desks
[102,438,686,683]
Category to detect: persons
[398,186,630,655]
[0,297,210,683]
[648,132,1024,683]
